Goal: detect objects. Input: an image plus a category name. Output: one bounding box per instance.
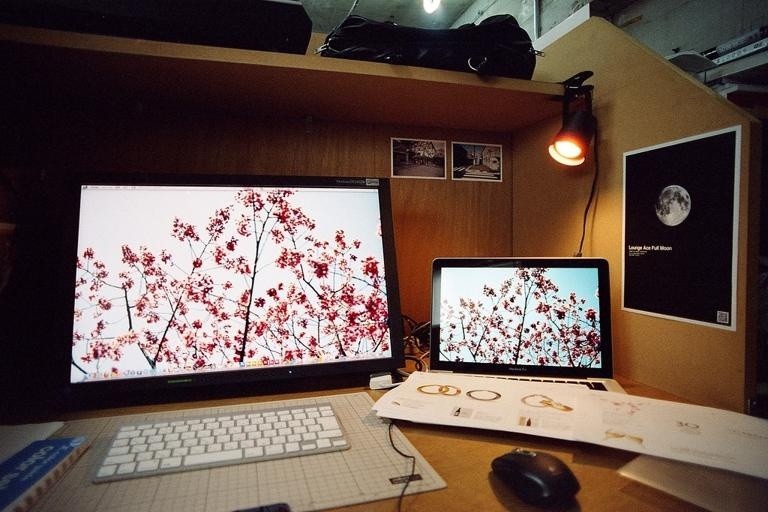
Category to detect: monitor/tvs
[57,171,406,401]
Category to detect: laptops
[429,256,627,396]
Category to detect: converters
[368,369,393,390]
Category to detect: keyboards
[92,401,351,484]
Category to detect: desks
[0,3,762,512]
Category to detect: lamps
[548,71,597,167]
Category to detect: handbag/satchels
[321,13,536,80]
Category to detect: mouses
[491,448,579,505]
